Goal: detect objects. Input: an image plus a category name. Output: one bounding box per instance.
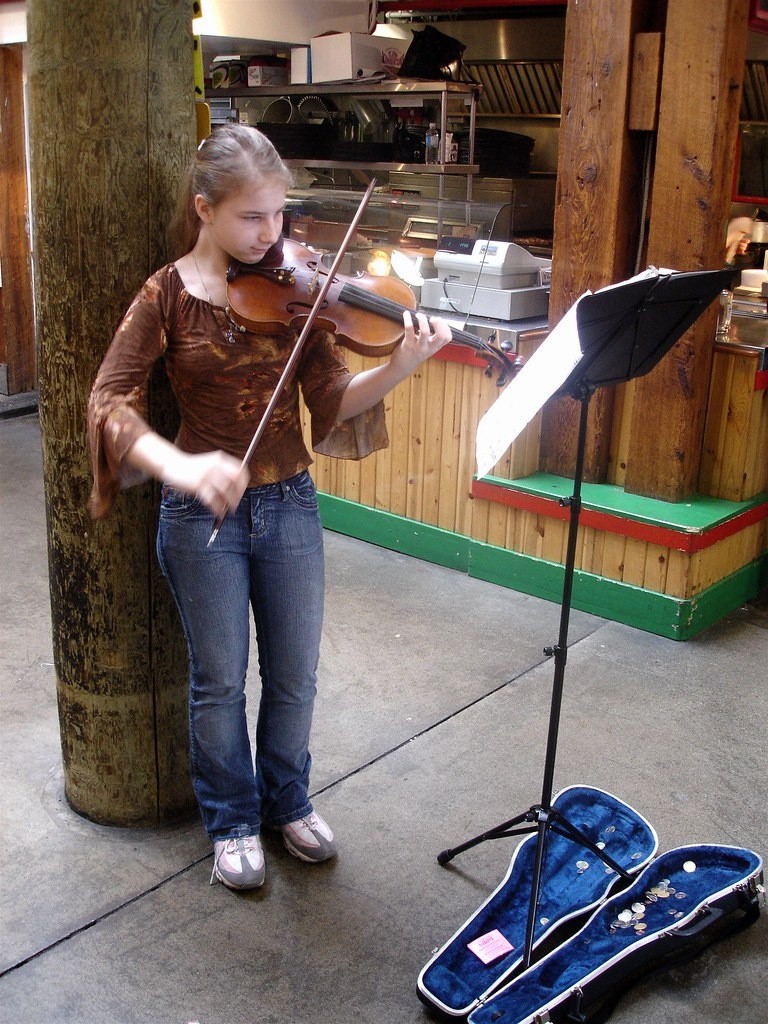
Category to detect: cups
[716,290,733,334]
[451,143,458,162]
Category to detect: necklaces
[190,249,236,343]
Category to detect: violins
[222,234,528,386]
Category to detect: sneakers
[281,810,338,863]
[210,833,266,890]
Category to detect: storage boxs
[210,54,249,90]
[247,62,291,87]
[290,29,410,84]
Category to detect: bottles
[425,123,439,166]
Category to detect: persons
[86,125,454,891]
